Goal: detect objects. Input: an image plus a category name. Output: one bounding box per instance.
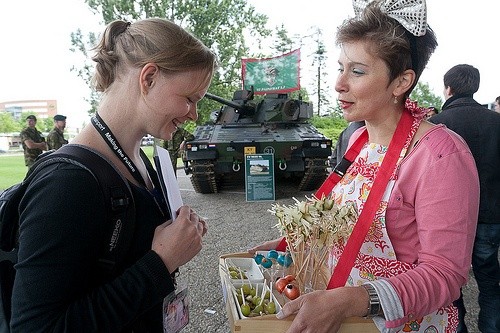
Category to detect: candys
[275,274,299,301]
[228,266,248,279]
[235,285,276,316]
[255,249,291,268]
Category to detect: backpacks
[0,144,134,333]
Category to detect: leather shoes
[185,167,190,175]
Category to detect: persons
[19,115,50,170]
[47,114,69,151]
[163,126,195,181]
[329,64,500,333]
[0,18,216,333]
[276,0,481,332]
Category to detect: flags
[241,48,301,93]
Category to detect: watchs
[361,284,381,320]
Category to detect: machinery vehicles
[177,85,333,194]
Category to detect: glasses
[427,108,435,113]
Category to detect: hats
[26,115,37,121]
[53,115,67,120]
